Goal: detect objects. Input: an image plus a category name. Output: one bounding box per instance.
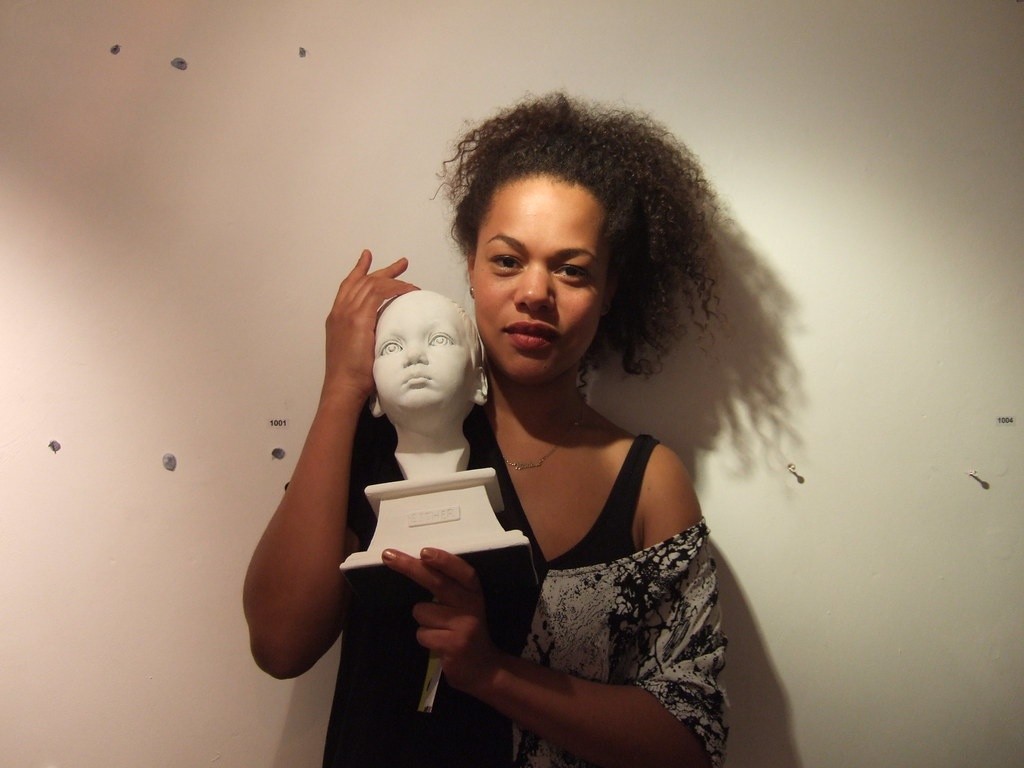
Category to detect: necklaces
[501,392,587,469]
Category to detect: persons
[243,92,732,768]
[339,290,540,482]
